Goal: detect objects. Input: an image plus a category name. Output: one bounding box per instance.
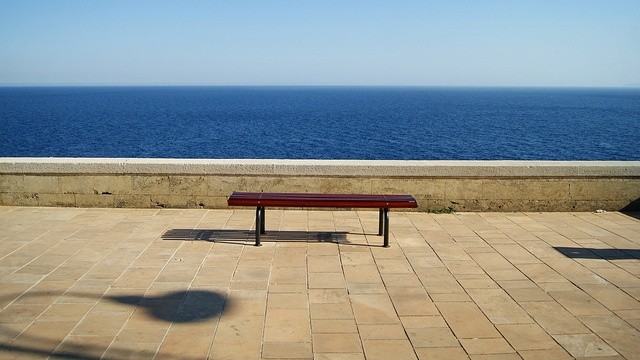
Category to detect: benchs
[227,192,418,247]
